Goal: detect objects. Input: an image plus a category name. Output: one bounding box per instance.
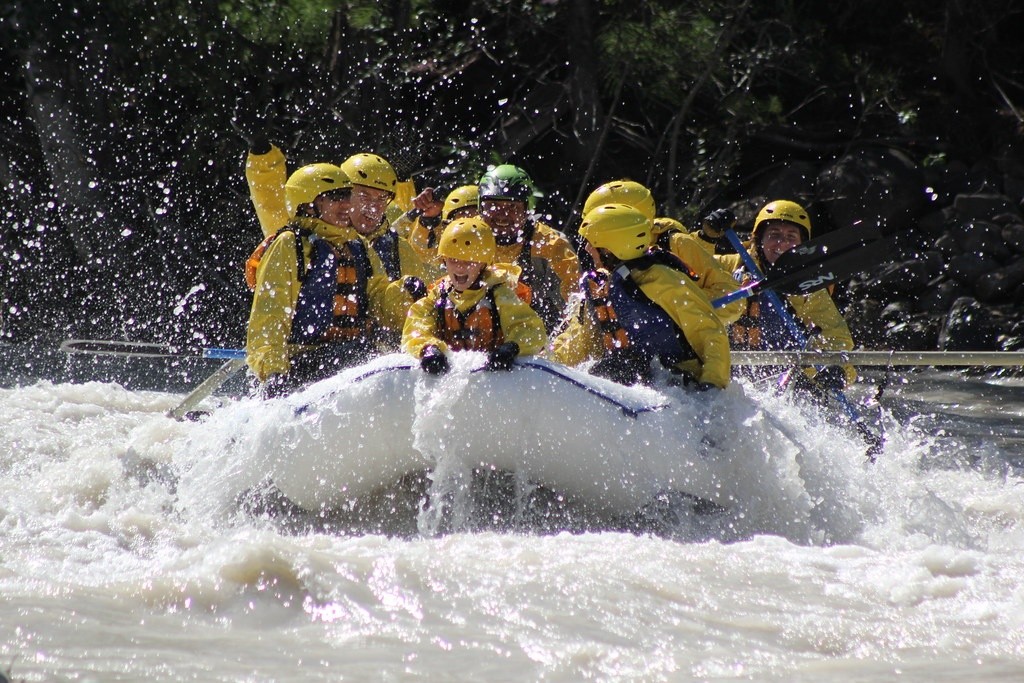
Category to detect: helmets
[438,218,495,266]
[341,153,397,199]
[752,200,811,242]
[478,164,532,216]
[581,181,656,230]
[284,163,354,219]
[577,203,651,260]
[442,185,478,219]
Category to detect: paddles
[61,339,246,359]
[730,350,1024,367]
[719,219,881,453]
[710,218,894,309]
[167,183,448,420]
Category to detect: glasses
[318,191,351,201]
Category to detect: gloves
[699,208,738,244]
[262,372,288,401]
[422,344,451,376]
[489,342,520,372]
[230,91,273,154]
[812,365,847,392]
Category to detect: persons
[229,90,859,408]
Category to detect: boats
[266,348,731,518]
